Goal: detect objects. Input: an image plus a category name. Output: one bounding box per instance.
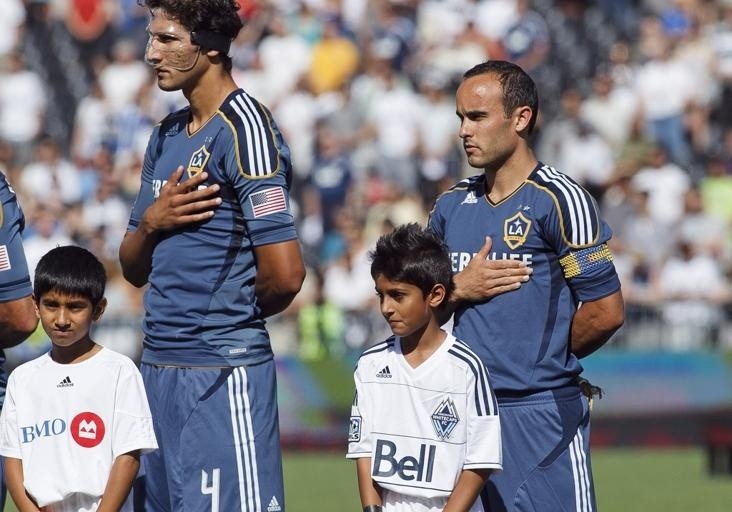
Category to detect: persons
[119,0,307,511]
[1,1,730,358]
[0,242,162,512]
[425,62,625,512]
[345,223,504,511]
[1,170,40,512]
